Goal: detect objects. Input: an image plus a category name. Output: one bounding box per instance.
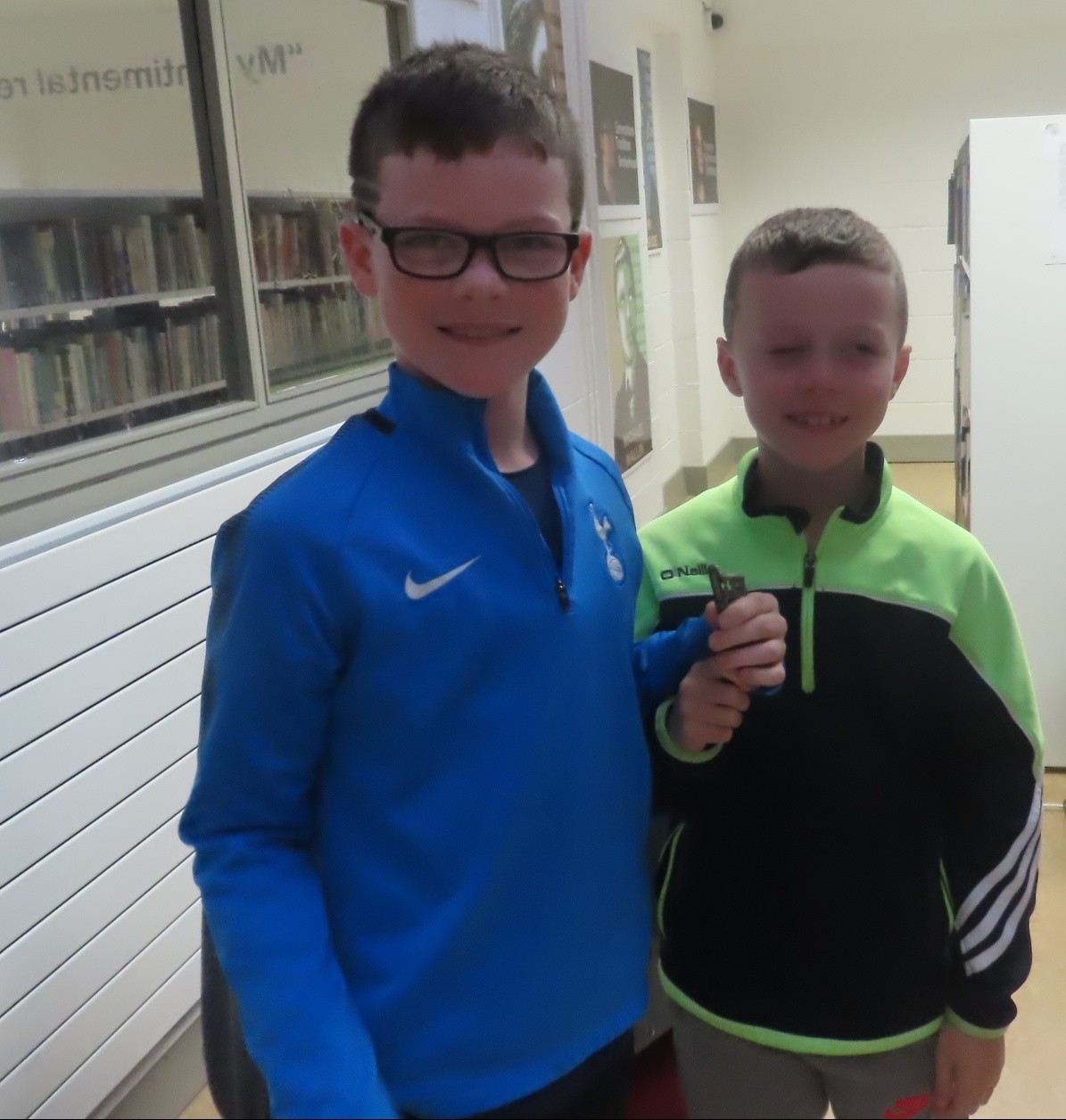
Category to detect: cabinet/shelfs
[946,116,1066,770]
[1,191,383,458]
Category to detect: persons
[176,44,786,1120]
[632,207,1046,1118]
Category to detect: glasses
[354,210,584,285]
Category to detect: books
[1,198,396,434]
